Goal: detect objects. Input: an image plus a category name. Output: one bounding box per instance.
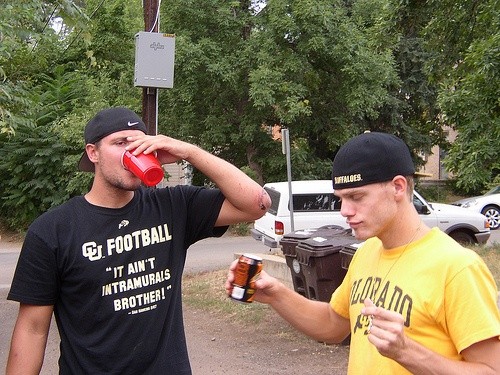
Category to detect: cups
[121,147,164,187]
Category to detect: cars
[452,176,500,230]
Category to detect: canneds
[229,254,263,303]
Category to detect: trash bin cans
[295,236,342,303]
[339,240,365,285]
[279,229,330,300]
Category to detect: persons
[226,129,500,375]
[4,105,271,375]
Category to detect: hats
[78,108,147,172]
[332,130,432,196]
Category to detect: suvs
[252,178,491,251]
